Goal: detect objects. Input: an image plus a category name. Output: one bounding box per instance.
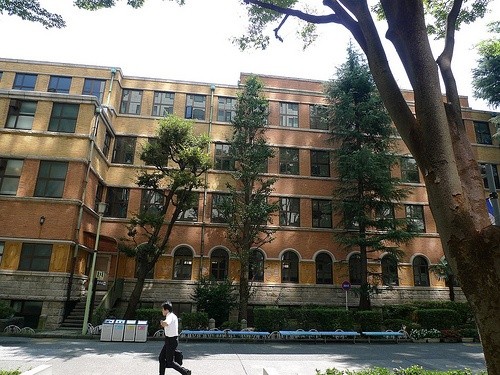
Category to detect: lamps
[40,216,45,225]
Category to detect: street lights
[82,201,110,336]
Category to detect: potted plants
[459,328,478,342]
[441,330,460,343]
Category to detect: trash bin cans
[100,319,115,342]
[135,320,148,342]
[112,320,126,341]
[124,320,136,342]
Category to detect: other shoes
[187,371,191,375]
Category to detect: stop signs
[341,280,351,291]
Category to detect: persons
[158,302,191,375]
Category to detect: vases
[412,338,427,343]
[428,338,440,343]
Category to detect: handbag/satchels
[174,349,183,365]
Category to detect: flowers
[409,328,442,340]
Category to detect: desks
[181,330,270,343]
[362,332,405,344]
[279,330,361,344]
